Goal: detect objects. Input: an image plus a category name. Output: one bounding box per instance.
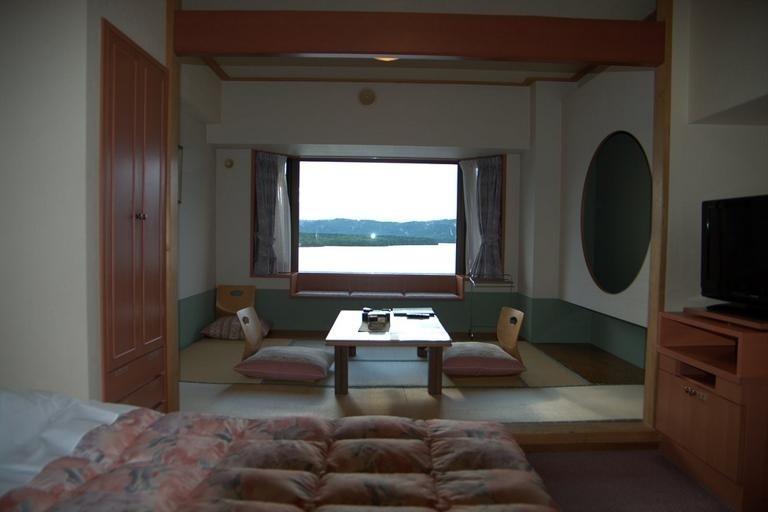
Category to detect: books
[392,306,435,317]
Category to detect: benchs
[288,271,465,302]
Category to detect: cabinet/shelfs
[101,16,168,415]
[655,312,768,511]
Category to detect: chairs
[442,303,528,377]
[200,283,296,360]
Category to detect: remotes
[404,313,430,317]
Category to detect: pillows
[230,344,336,385]
[443,338,524,383]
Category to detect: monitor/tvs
[701,194,768,322]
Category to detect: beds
[0,393,558,510]
